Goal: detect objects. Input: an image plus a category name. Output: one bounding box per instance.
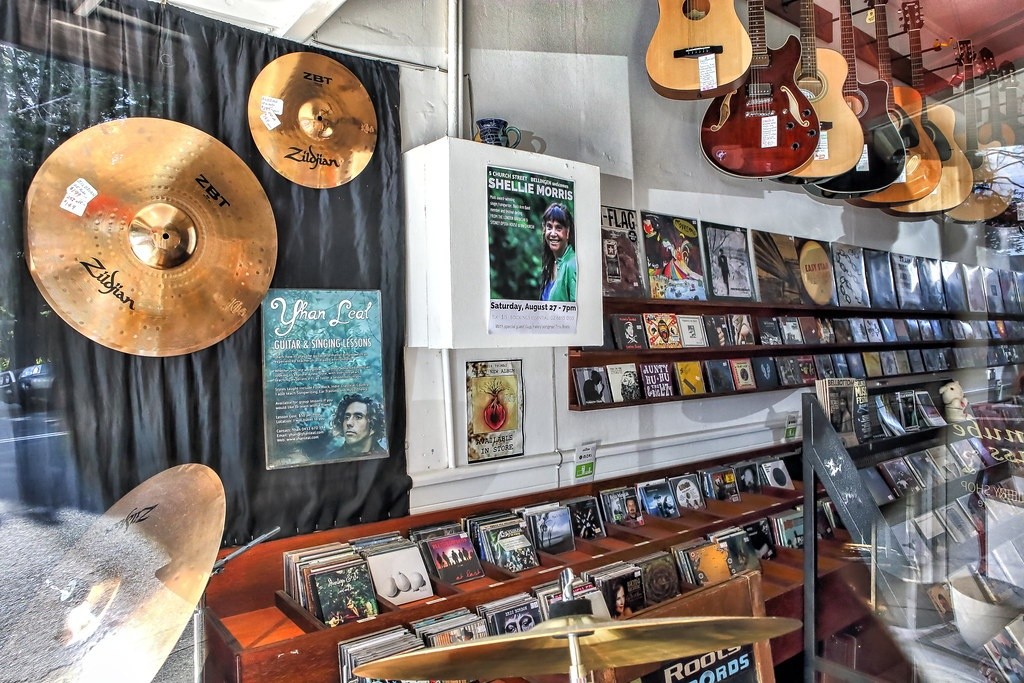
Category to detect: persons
[457,629,473,642]
[623,498,642,529]
[839,391,852,433]
[319,393,386,460]
[938,594,954,621]
[621,322,638,345]
[538,203,577,302]
[607,577,632,620]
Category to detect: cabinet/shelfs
[801,379,1024,683]
[198,416,1022,682]
[565,205,1024,408]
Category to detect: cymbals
[21,116,280,359]
[350,612,804,681]
[247,51,379,191]
[0,462,228,683]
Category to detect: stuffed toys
[939,381,969,422]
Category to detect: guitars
[799,0,907,201]
[768,1,864,184]
[879,0,975,218]
[843,0,943,208]
[976,46,1024,226]
[934,39,1014,225]
[697,0,821,180]
[645,1,754,100]
